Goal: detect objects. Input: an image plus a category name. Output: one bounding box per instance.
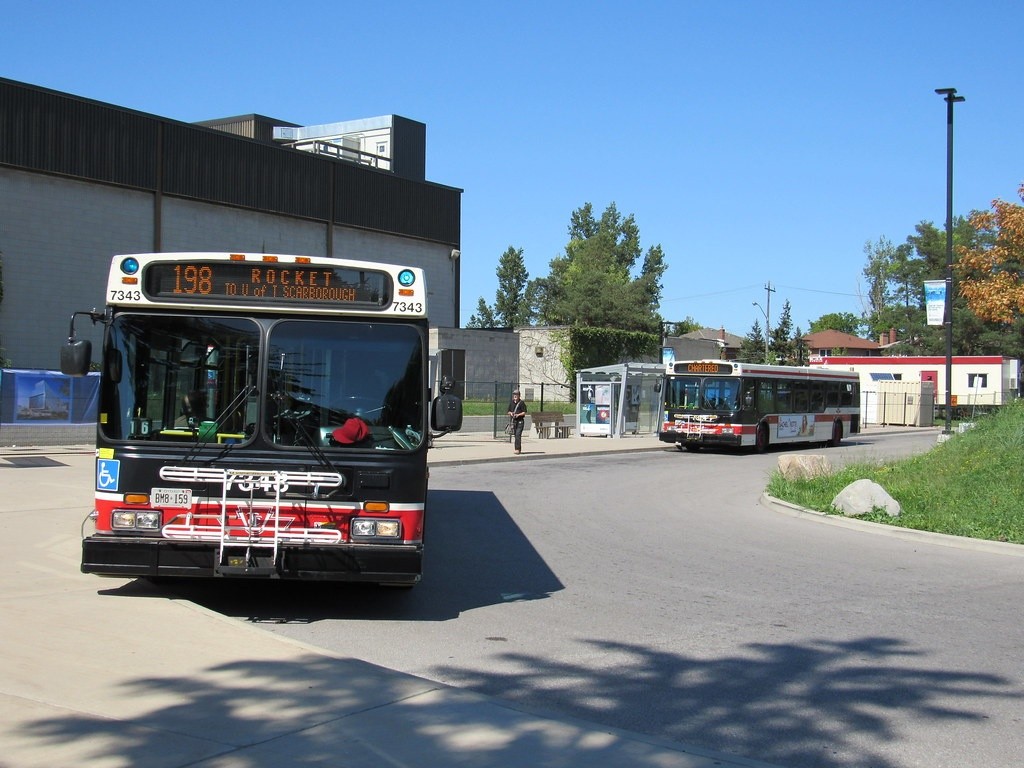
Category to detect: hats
[333,417,369,444]
[512,390,520,395]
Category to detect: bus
[653,358,862,454]
[57,250,463,595]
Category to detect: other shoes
[514,449,521,453]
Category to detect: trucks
[806,353,1022,420]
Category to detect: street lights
[751,301,771,365]
[936,87,970,433]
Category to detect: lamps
[450,249,461,260]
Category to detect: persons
[507,390,527,454]
[181,394,196,415]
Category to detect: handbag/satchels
[504,421,516,435]
